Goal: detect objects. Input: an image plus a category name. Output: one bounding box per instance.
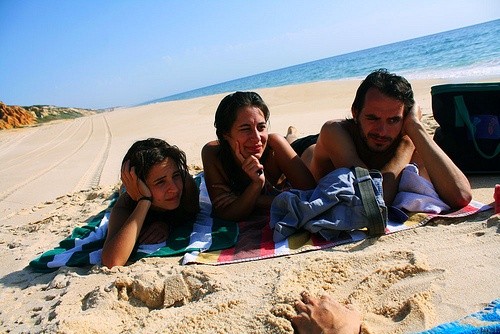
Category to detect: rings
[256,169,263,175]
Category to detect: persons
[201,92,316,222]
[101,138,200,269]
[286,68,472,209]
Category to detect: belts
[355,166,384,236]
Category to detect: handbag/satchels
[431,82,500,175]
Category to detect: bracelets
[137,197,153,204]
